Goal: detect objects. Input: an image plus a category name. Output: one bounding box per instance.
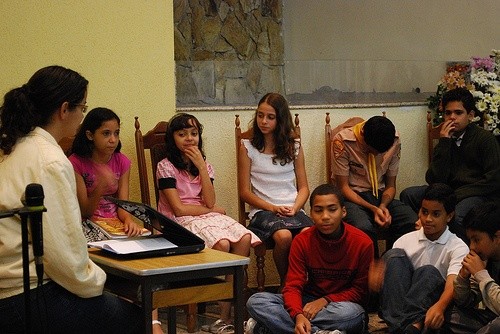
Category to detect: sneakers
[243,318,272,334]
[312,329,343,334]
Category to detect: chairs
[135,116,248,334]
[235,113,307,290]
[427,110,489,162]
[325,111,390,260]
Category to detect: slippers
[201,318,234,334]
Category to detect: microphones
[25,183,46,285]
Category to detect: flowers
[424,49,500,136]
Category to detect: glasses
[70,103,89,113]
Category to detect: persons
[247,183,374,334]
[156,113,262,324]
[0,66,164,334]
[331,87,500,334]
[237,92,318,290]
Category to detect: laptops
[99,196,206,259]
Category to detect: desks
[88,247,251,334]
